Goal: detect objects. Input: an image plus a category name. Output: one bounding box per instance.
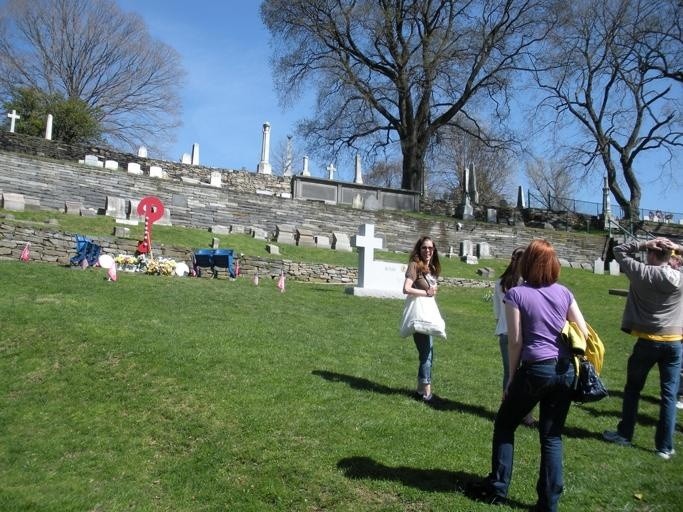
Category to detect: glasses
[419,246,432,250]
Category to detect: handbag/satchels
[399,295,446,340]
[568,359,608,403]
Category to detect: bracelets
[426,290,430,296]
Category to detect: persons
[491,246,545,429]
[402,235,441,403]
[598,236,682,458]
[664,245,682,412]
[474,238,590,512]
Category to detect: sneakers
[653,446,676,460]
[602,428,631,448]
[461,478,503,502]
[415,391,431,402]
[522,413,537,427]
